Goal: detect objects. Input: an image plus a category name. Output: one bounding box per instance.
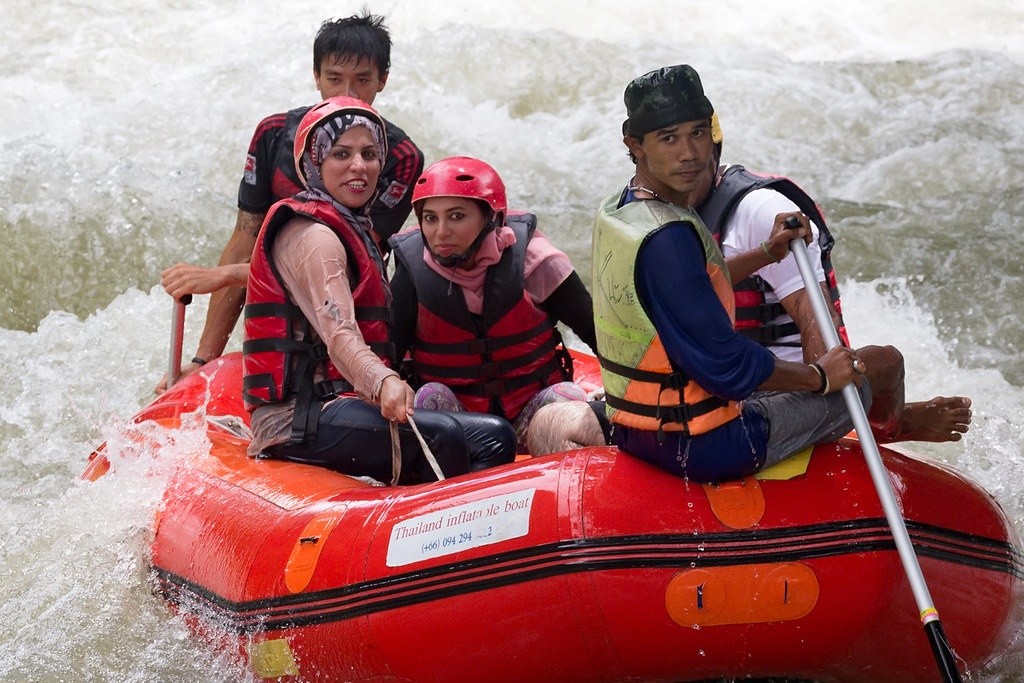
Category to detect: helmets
[294,96,388,194]
[708,109,723,144]
[410,157,507,241]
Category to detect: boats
[147,345,1024,683]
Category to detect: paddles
[783,213,960,683]
[165,292,195,389]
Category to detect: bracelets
[807,364,831,396]
[192,357,207,366]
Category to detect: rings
[388,415,397,422]
[852,359,868,376]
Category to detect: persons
[243,96,518,485]
[591,65,972,488]
[155,5,424,399]
[528,97,852,459]
[391,156,597,453]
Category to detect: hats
[624,64,714,137]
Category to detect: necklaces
[629,186,680,207]
